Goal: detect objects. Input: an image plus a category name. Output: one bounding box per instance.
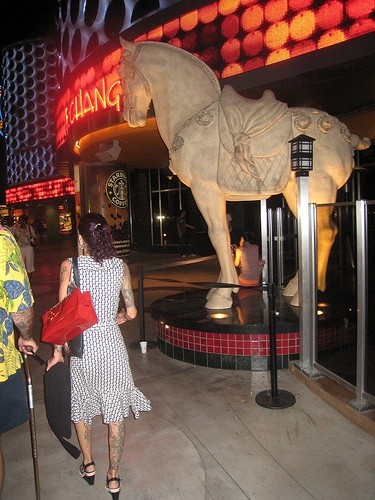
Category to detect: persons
[234,231,261,287]
[226,213,233,246]
[0,213,39,498]
[177,209,200,258]
[46,213,152,500]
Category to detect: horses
[118,36,372,309]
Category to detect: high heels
[78,460,96,485]
[105,474,120,500]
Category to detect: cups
[140,341,147,353]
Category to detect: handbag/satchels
[28,225,35,246]
[42,257,97,344]
